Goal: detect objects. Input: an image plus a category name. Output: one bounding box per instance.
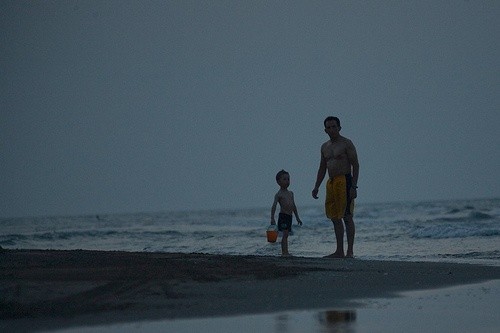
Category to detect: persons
[311,115,360,261]
[270,169,302,258]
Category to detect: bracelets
[349,184,358,192]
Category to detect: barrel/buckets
[266,225,279,242]
